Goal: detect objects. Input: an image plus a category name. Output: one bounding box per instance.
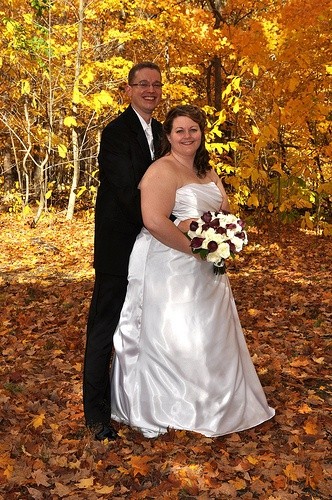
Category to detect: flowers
[187,210,248,275]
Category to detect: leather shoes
[93,421,118,440]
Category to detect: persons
[141,105,231,432]
[82,60,202,440]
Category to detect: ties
[146,125,157,165]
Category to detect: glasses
[129,80,165,91]
